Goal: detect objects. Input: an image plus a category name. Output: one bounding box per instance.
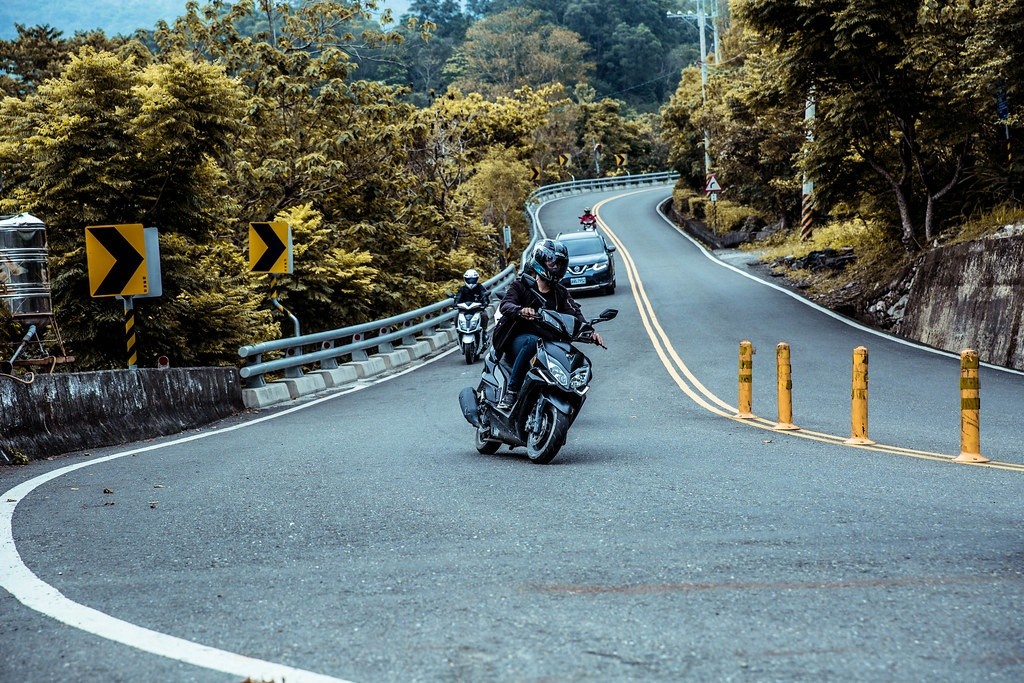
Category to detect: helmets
[527,238,569,283]
[584,207,591,212]
[462,269,479,290]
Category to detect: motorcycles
[458,273,619,466]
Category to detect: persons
[453,270,491,344]
[492,238,604,410]
[580,208,596,230]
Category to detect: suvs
[555,232,616,297]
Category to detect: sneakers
[498,390,517,411]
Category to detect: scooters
[578,214,598,232]
[446,291,492,364]
